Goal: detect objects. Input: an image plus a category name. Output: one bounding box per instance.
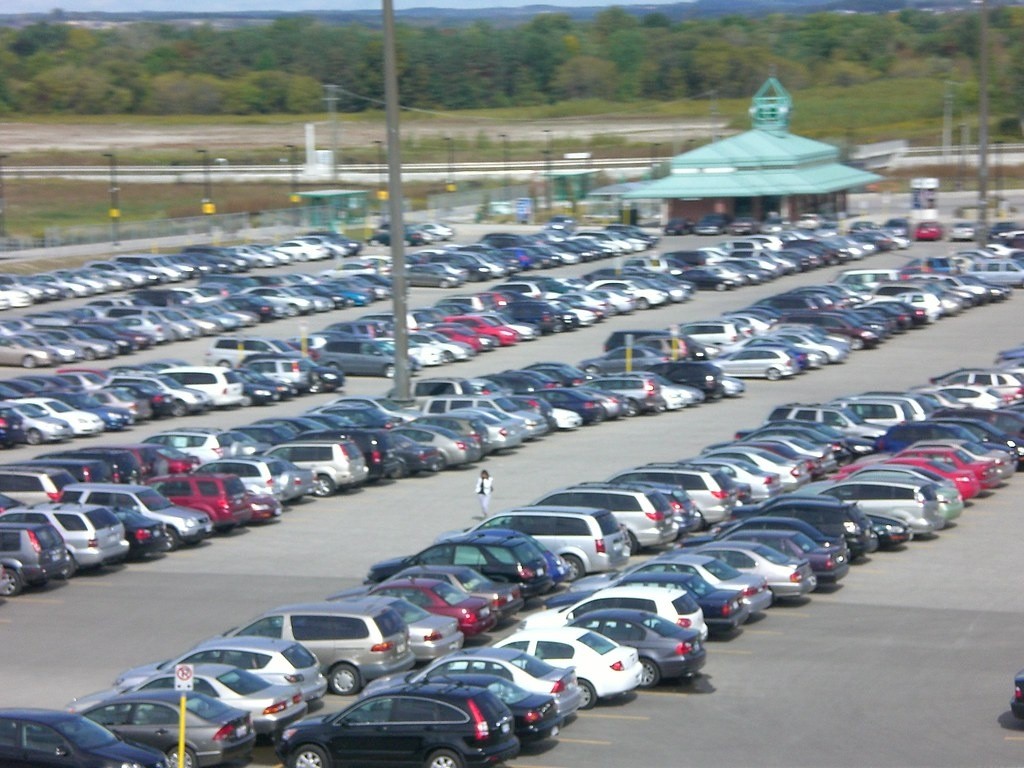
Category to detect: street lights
[541,125,551,209]
[372,138,385,215]
[102,152,119,244]
[196,148,213,237]
[443,137,455,211]
[286,144,300,228]
[495,132,508,200]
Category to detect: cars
[0,214,1024,598]
[2,349,1023,768]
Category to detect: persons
[476,470,493,518]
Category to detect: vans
[440,504,632,583]
[156,365,246,407]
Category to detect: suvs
[525,485,679,552]
[765,403,889,439]
